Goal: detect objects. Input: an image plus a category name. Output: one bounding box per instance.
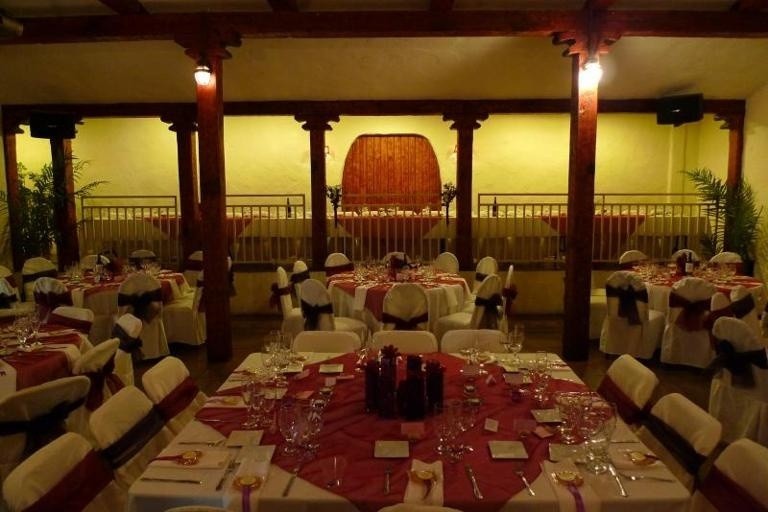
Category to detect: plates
[235,332,663,508]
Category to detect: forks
[206,337,665,505]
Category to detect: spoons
[172,338,673,499]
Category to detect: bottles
[94,254,105,277]
[683,251,693,276]
[401,253,410,281]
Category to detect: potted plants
[0,144,113,260]
[674,166,768,277]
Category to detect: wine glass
[159,346,676,505]
[9,308,43,354]
